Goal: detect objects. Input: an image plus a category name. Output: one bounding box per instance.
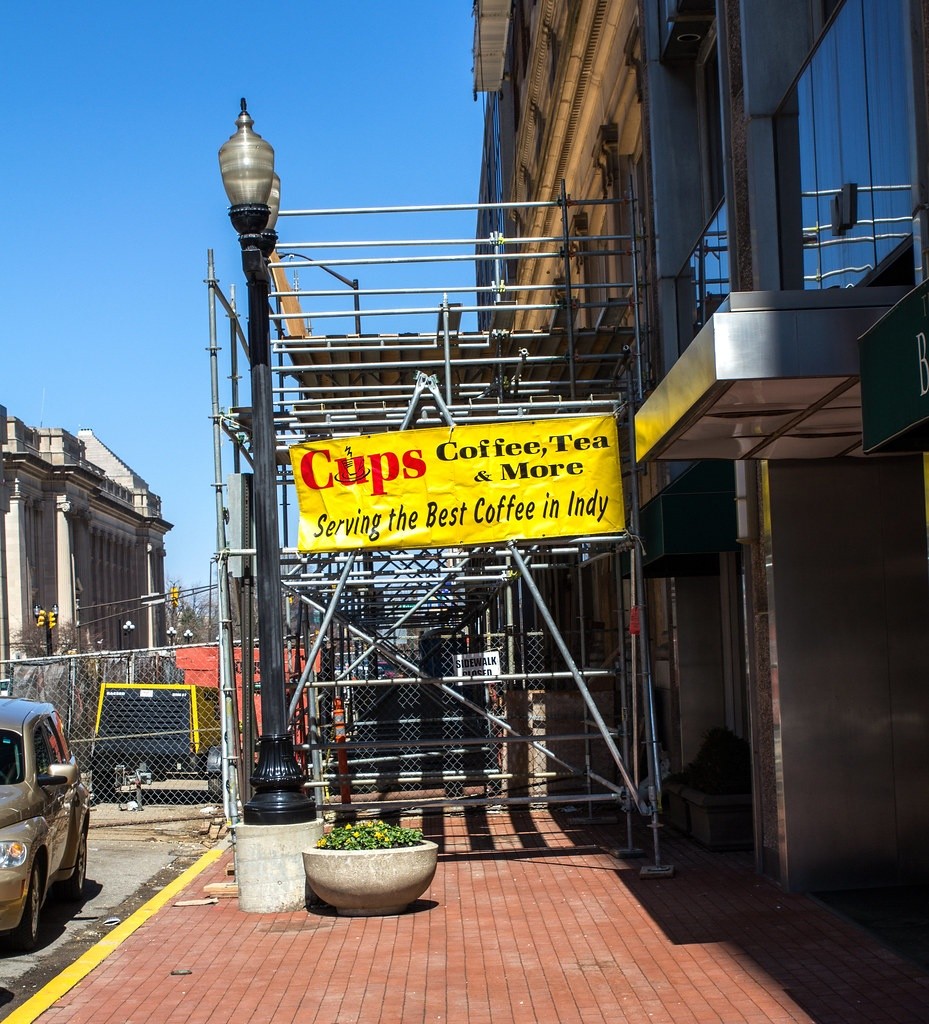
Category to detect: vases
[302,842,440,916]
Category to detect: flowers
[316,821,422,850]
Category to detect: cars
[0,694,92,950]
[0,679,10,696]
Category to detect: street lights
[166,626,177,646]
[33,603,59,657]
[183,629,193,644]
[123,620,136,649]
[215,95,319,824]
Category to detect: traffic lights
[48,612,56,629]
[38,610,46,626]
